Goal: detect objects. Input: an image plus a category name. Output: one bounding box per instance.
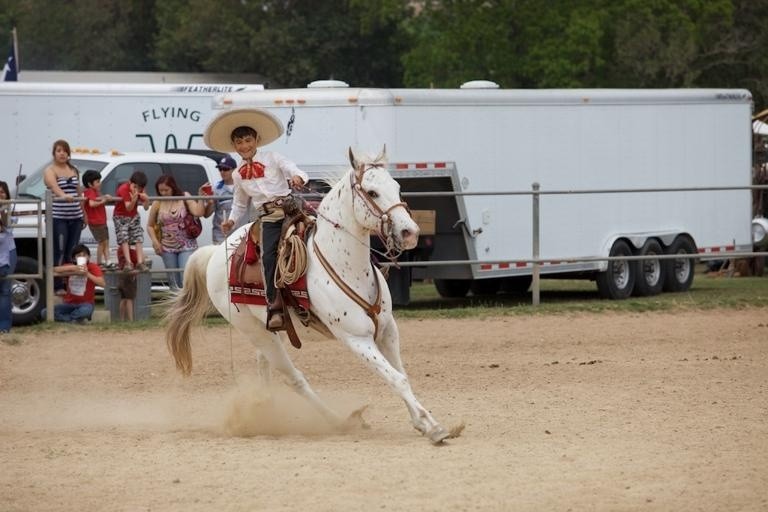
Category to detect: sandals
[98,262,119,271]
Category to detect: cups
[76,256,88,273]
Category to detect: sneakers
[123,262,149,272]
[268,311,283,332]
[75,318,89,325]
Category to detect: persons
[44,140,88,295]
[0,181,18,232]
[113,172,149,272]
[40,244,106,324]
[221,126,309,330]
[204,158,249,245]
[117,243,137,320]
[0,218,10,334]
[82,170,115,268]
[147,176,211,290]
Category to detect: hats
[215,157,237,168]
[206,109,281,152]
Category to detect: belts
[257,197,295,212]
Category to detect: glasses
[219,166,233,171]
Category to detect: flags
[1,32,17,81]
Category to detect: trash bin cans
[103,265,150,321]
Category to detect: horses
[156,142,452,447]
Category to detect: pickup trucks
[1,152,437,328]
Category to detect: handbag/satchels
[184,214,202,239]
